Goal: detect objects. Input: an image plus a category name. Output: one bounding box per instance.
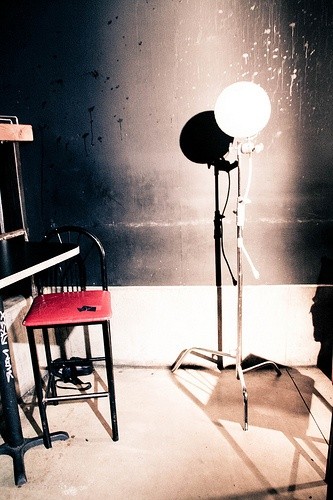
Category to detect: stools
[22,226,119,450]
